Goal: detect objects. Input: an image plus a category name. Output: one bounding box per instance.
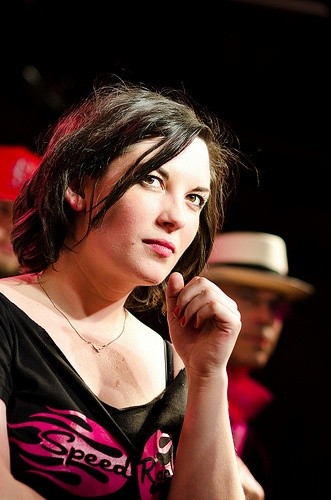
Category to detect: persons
[0,82,245,500]
[0,147,311,500]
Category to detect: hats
[0,145,43,202]
[198,232,314,301]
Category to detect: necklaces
[37,274,129,352]
